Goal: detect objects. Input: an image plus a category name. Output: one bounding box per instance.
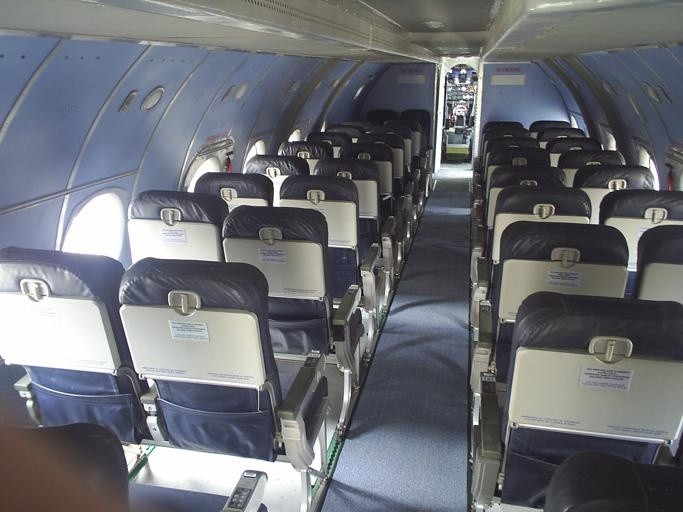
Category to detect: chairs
[541,448,683,511]
[126,109,434,436]
[466,119,683,432]
[1,422,311,512]
[0,246,145,443]
[469,290,683,511]
[118,258,336,481]
